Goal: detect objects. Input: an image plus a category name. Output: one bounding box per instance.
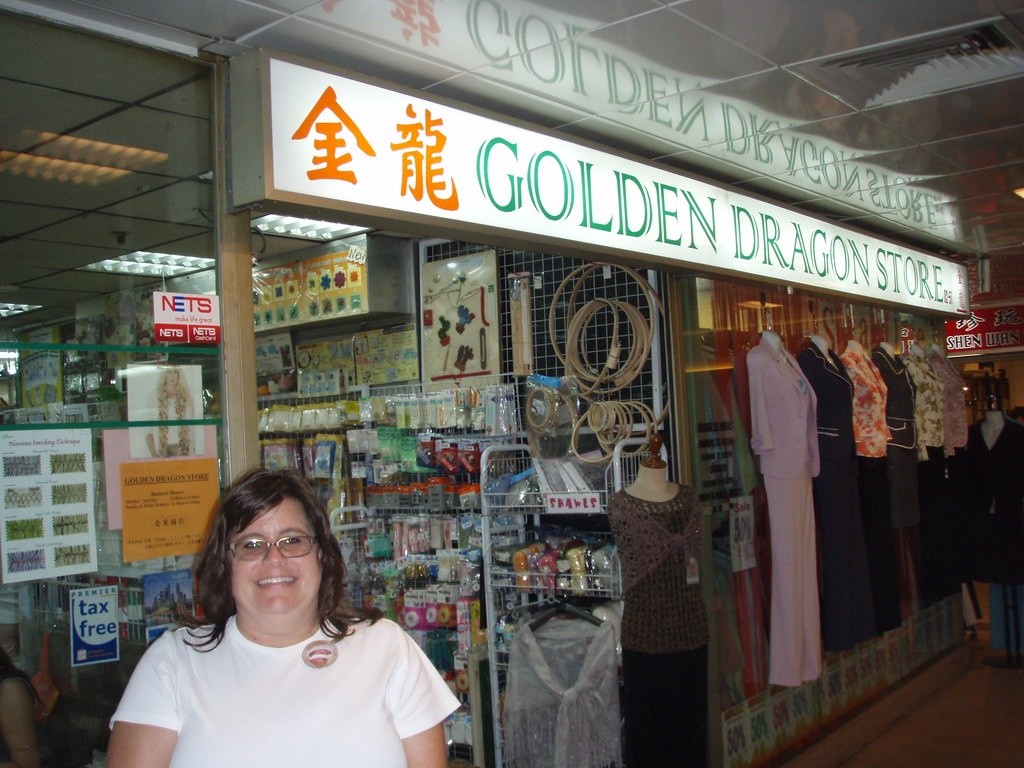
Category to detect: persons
[107,467,463,768]
[139,366,194,458]
[605,435,709,768]
[747,330,1024,688]
[0,647,48,768]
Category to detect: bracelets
[8,744,38,754]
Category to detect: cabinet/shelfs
[481,437,669,768]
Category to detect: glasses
[226,535,319,561]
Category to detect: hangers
[512,572,604,631]
